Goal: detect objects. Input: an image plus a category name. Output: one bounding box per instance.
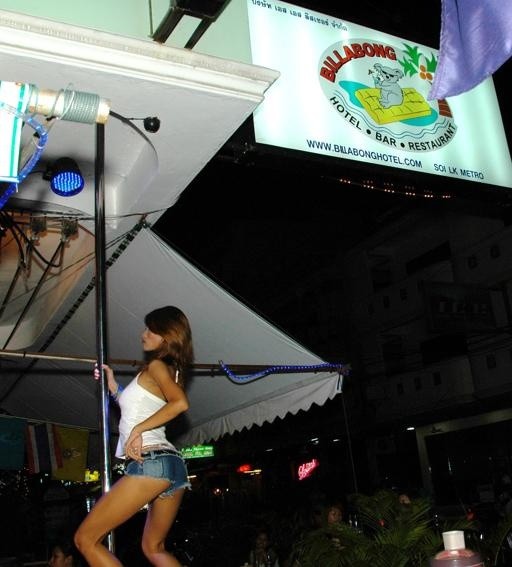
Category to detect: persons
[323,505,343,526]
[73,305,196,567]
[397,494,411,504]
[247,530,277,566]
[47,543,80,567]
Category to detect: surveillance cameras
[143,116,160,133]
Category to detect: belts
[125,449,182,471]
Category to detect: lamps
[40,153,87,199]
